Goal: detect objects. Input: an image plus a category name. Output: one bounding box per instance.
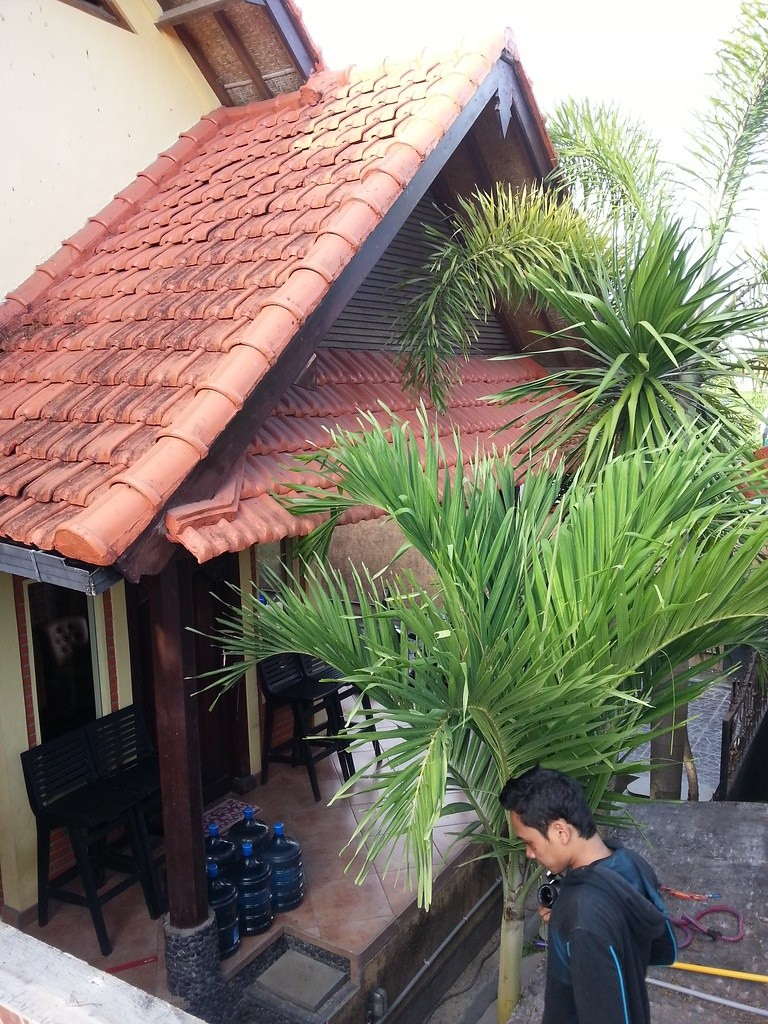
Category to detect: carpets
[202,798,263,838]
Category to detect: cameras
[538,870,564,908]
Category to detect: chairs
[21,705,167,957]
[257,653,383,802]
[37,615,95,723]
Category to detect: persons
[500,767,677,1024]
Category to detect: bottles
[226,842,273,935]
[206,823,240,884]
[207,864,240,960]
[229,807,269,861]
[258,822,304,913]
